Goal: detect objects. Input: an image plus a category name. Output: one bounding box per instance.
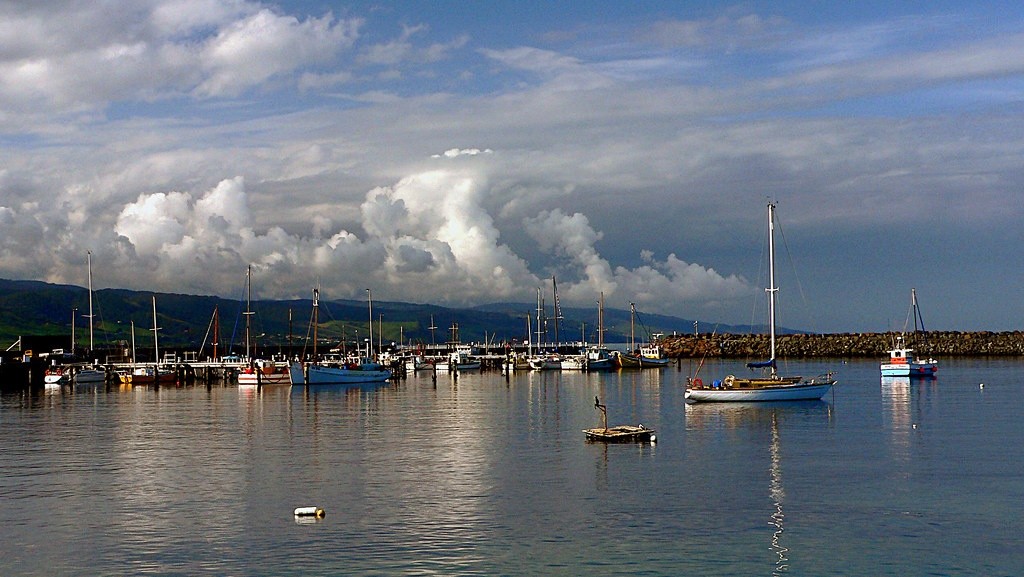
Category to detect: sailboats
[0,248,670,387]
[880,287,938,378]
[684,201,838,403]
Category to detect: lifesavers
[691,378,704,387]
[45,369,53,376]
[56,369,62,376]
